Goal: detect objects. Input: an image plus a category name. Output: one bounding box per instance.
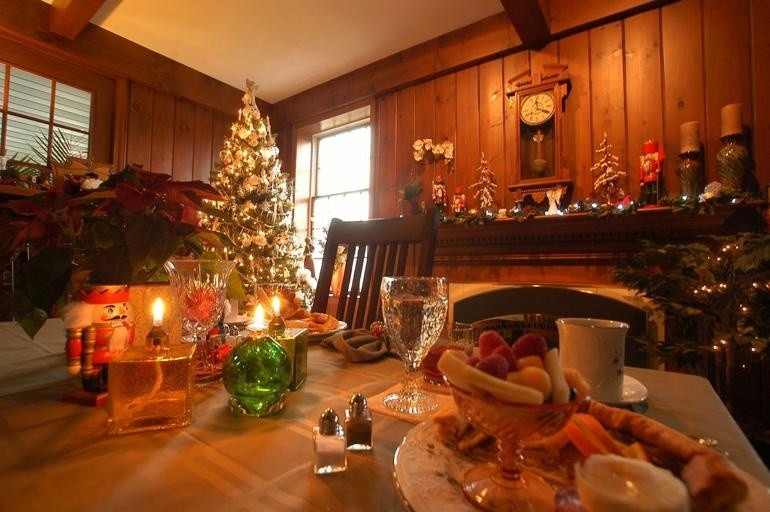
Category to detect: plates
[608,374,648,406]
[392,403,770,511]
[306,318,349,343]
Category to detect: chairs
[311,208,440,331]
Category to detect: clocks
[506,71,573,203]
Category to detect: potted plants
[398,178,424,216]
[0,158,248,343]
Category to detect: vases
[675,153,703,197]
[716,134,749,191]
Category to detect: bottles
[341,393,374,451]
[312,408,348,474]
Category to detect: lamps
[103,294,308,436]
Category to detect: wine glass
[442,368,580,512]
[377,274,450,414]
[163,257,238,384]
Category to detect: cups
[553,317,629,401]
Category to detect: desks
[0,318,770,512]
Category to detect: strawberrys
[467,331,548,377]
